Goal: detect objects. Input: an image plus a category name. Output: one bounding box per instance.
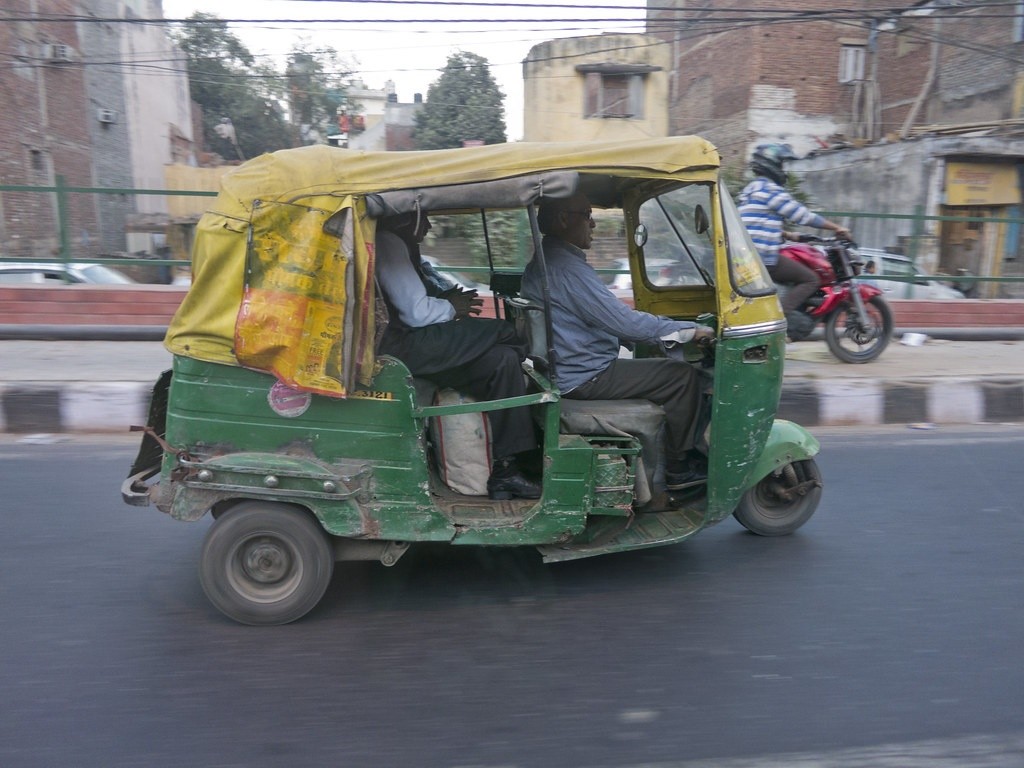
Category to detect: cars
[0,262,138,285]
[600,256,703,299]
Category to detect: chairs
[509,297,668,512]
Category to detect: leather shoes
[487,471,541,500]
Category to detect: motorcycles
[658,233,893,366]
[121,134,822,627]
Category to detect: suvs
[812,244,965,299]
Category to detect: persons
[940,270,963,292]
[522,193,714,490]
[864,261,877,286]
[737,144,851,344]
[374,211,540,500]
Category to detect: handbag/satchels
[428,385,494,496]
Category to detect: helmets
[751,144,800,185]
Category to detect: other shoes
[665,460,709,488]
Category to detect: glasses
[565,211,592,220]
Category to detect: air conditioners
[49,44,75,64]
[100,109,119,125]
